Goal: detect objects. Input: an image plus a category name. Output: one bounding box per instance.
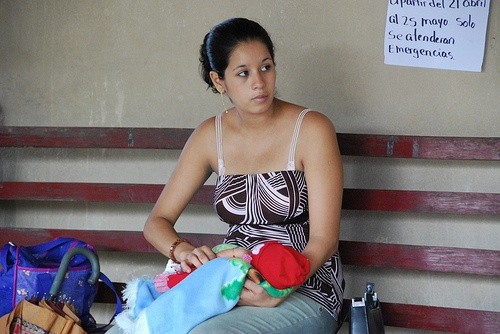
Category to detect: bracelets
[169,239,192,264]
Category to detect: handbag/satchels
[0,236,122,332]
[350,282,385,334]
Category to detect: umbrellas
[0,247,100,334]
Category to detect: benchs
[0,126,500,334]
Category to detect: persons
[104,18,346,334]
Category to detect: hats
[251,241,311,289]
